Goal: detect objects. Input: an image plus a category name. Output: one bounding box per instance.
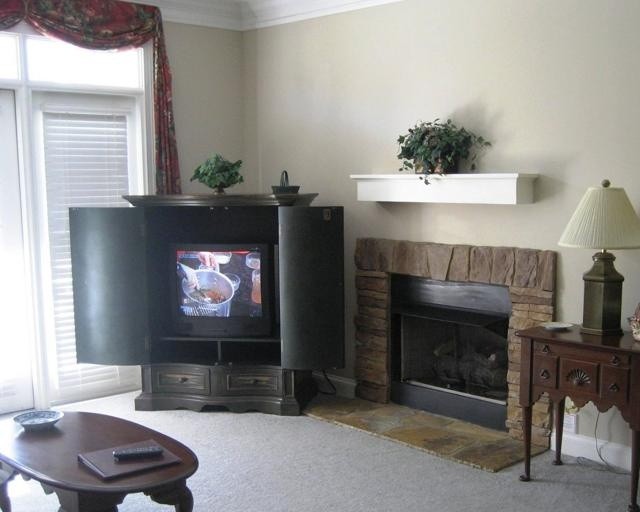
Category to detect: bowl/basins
[198,264,219,272]
[245,252,261,269]
[224,273,241,292]
[212,251,232,265]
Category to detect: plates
[538,322,574,331]
[12,409,65,432]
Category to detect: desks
[515,323,640,512]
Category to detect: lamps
[558,180,640,335]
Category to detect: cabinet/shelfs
[72,194,344,415]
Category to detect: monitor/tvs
[169,242,271,337]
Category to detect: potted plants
[397,119,492,185]
[190,155,244,194]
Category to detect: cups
[251,269,262,305]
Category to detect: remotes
[113,446,163,459]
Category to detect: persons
[177,251,218,291]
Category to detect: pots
[181,265,234,317]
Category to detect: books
[78,439,184,480]
